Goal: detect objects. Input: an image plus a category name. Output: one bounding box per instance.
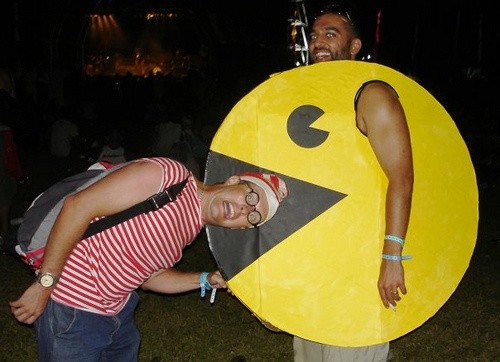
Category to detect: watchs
[37,272,60,288]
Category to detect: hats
[237,173,288,229]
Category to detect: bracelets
[199,272,217,303]
[382,254,411,261]
[384,235,405,247]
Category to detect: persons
[294,9,415,362]
[44,94,185,166]
[0,121,27,247]
[9,157,289,362]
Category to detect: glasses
[241,180,261,228]
[313,4,353,23]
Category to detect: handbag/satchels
[16,161,191,272]
